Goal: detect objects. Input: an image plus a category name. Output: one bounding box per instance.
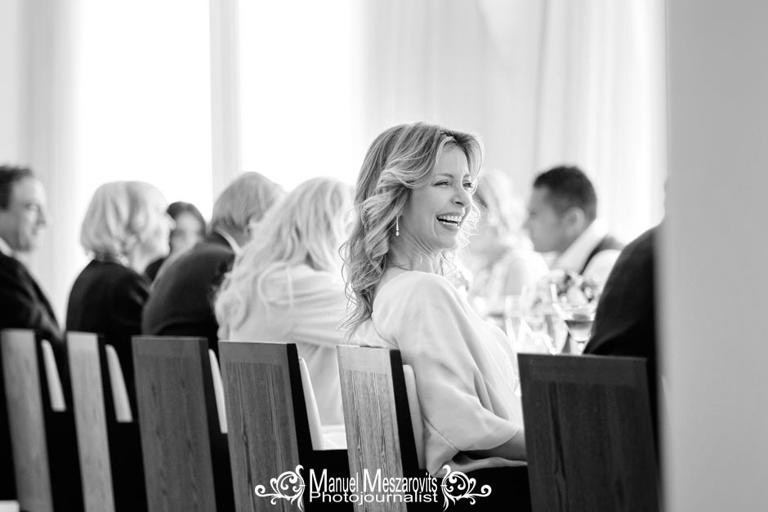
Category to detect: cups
[504,271,604,354]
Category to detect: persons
[143,201,209,289]
[521,163,627,353]
[345,119,538,512]
[64,177,154,340]
[574,219,664,476]
[446,176,548,323]
[204,176,371,512]
[146,169,285,346]
[0,166,67,343]
